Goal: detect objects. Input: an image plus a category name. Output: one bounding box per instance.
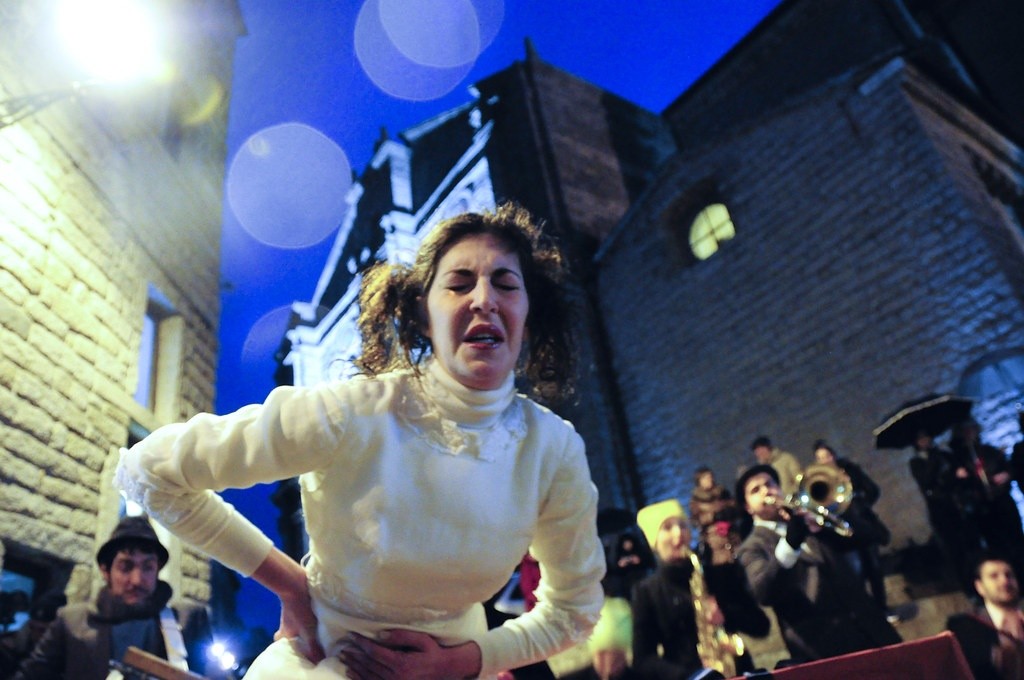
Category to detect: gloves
[785,513,812,551]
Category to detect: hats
[736,463,779,517]
[96,516,169,571]
[635,499,688,552]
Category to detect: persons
[946,557,1024,680]
[11,516,173,680]
[589,437,891,680]
[910,412,1024,595]
[112,203,607,680]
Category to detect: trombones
[763,462,857,538]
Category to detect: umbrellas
[871,392,974,451]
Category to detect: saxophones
[678,543,746,680]
